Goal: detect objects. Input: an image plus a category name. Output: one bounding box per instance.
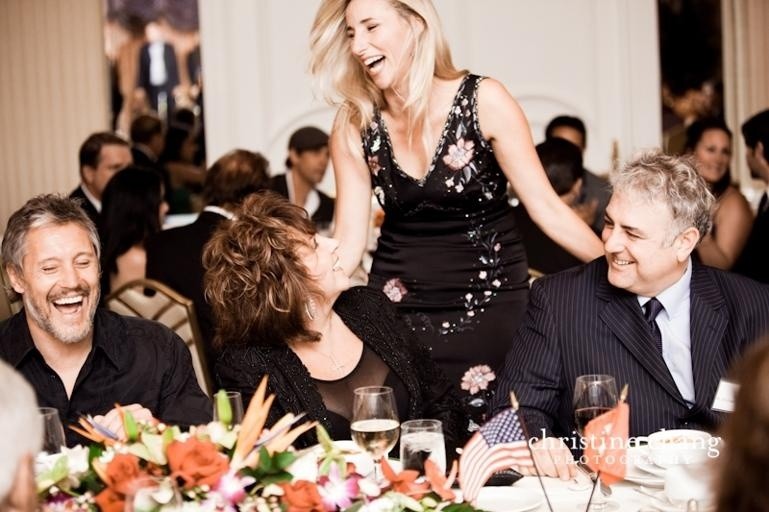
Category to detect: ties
[643,299,663,355]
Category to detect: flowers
[29,374,484,511]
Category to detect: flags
[458,406,534,508]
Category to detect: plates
[465,484,542,511]
[624,446,720,512]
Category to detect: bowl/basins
[648,428,714,466]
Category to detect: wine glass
[350,386,399,485]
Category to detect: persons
[200,186,468,490]
[661,78,719,136]
[486,146,768,481]
[0,190,214,456]
[0,358,45,512]
[310,0,607,440]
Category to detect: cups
[38,408,67,454]
[124,474,185,512]
[573,375,619,438]
[213,392,244,430]
[399,420,446,483]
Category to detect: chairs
[103,279,214,400]
